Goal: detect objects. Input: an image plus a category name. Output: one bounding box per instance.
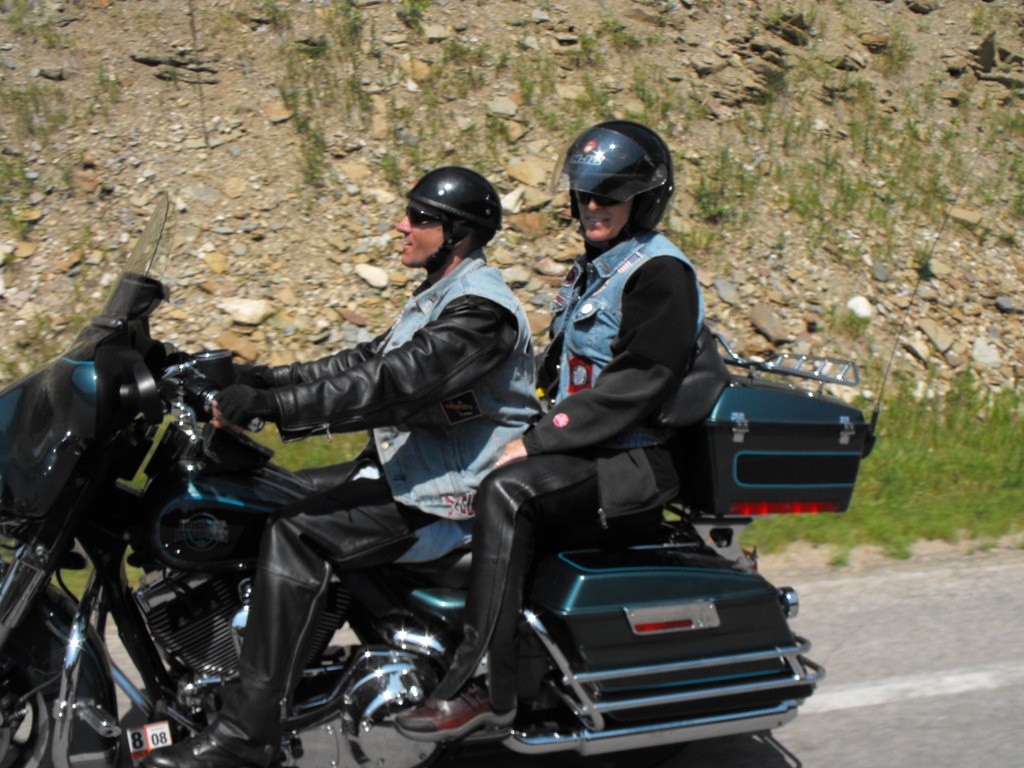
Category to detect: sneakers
[394,679,518,742]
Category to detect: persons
[140,166,543,768]
[395,118,702,742]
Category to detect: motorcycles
[2,192,877,768]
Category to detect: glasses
[406,205,440,227]
[573,188,626,206]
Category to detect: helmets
[404,166,503,230]
[561,120,674,231]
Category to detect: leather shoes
[140,731,277,768]
